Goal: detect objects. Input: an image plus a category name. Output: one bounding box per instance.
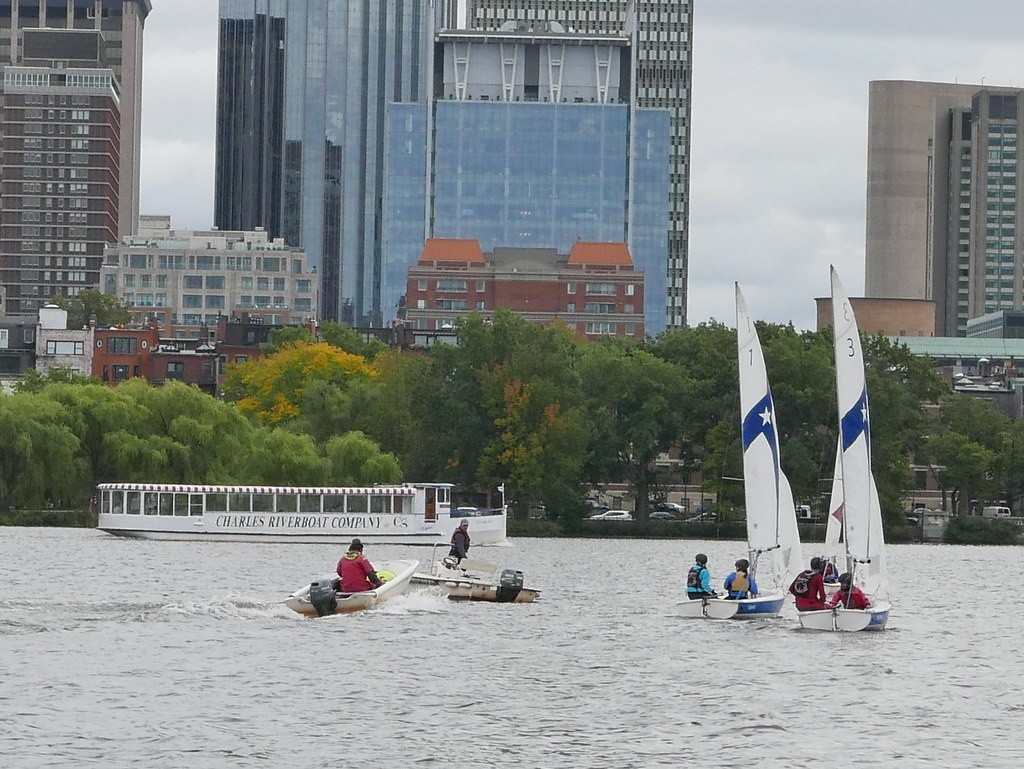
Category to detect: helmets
[838,573,852,582]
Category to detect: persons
[827,572,871,610]
[336,538,384,592]
[448,518,471,572]
[724,559,758,600]
[687,553,718,601]
[789,557,834,611]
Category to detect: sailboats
[676,280,807,619]
[797,265,891,634]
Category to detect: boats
[96,482,508,544]
[411,542,542,604]
[278,560,421,619]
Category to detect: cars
[446,495,1024,525]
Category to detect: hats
[461,519,469,526]
